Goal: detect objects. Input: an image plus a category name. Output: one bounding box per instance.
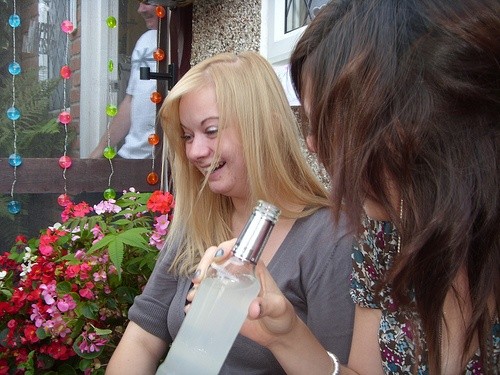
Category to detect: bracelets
[327,351,340,375]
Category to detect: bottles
[157,200,281,375]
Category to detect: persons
[104,52,355,375]
[91,2,158,159]
[183,0,500,375]
